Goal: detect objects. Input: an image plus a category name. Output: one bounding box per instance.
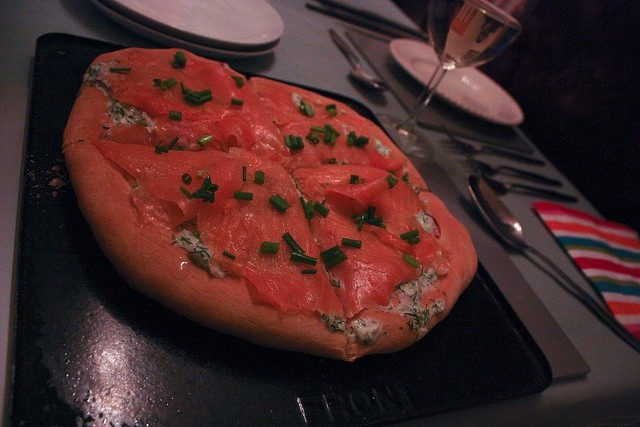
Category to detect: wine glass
[375,0,534,161]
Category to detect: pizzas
[60,46,479,365]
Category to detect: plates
[88,1,285,59]
[389,38,524,126]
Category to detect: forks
[480,166,577,205]
[442,122,546,166]
[459,155,562,188]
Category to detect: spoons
[466,172,640,353]
[328,25,392,92]
